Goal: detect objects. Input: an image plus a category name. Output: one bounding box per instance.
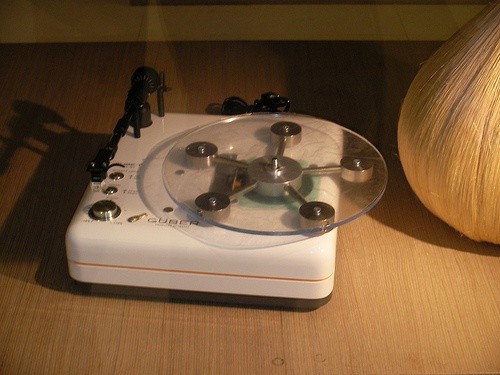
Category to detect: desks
[0,42,500,375]
